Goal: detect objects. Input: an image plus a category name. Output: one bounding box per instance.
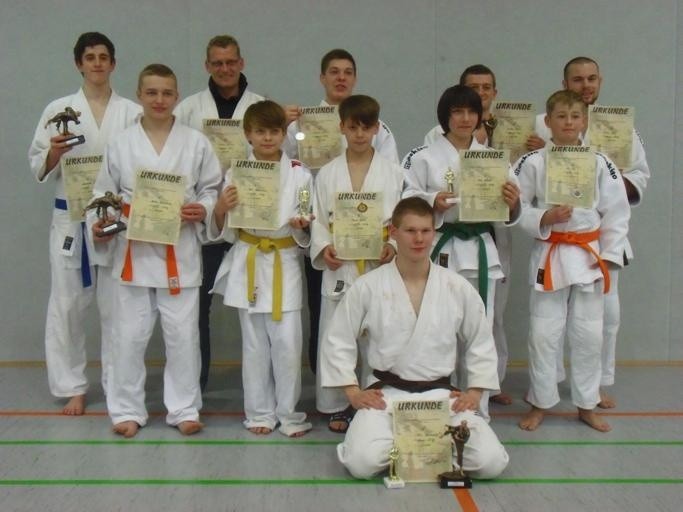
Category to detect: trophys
[44,107,85,147]
[86,191,127,237]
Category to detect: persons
[28,33,145,415]
[86,64,222,438]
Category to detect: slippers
[328,409,353,433]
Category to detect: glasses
[207,58,241,67]
